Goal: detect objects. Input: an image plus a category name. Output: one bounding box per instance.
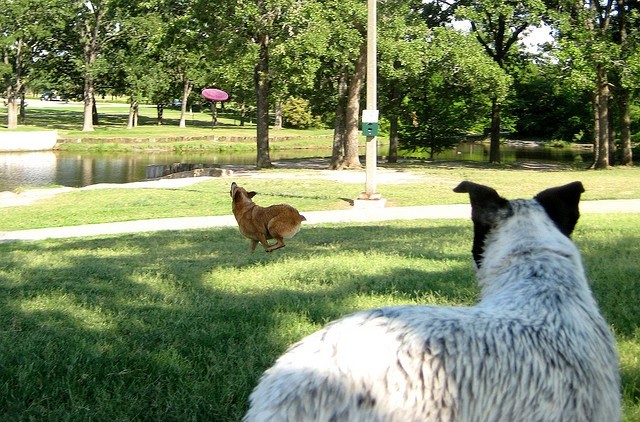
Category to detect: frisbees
[203,89,229,101]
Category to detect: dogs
[230,182,306,256]
[240,182,624,422]
